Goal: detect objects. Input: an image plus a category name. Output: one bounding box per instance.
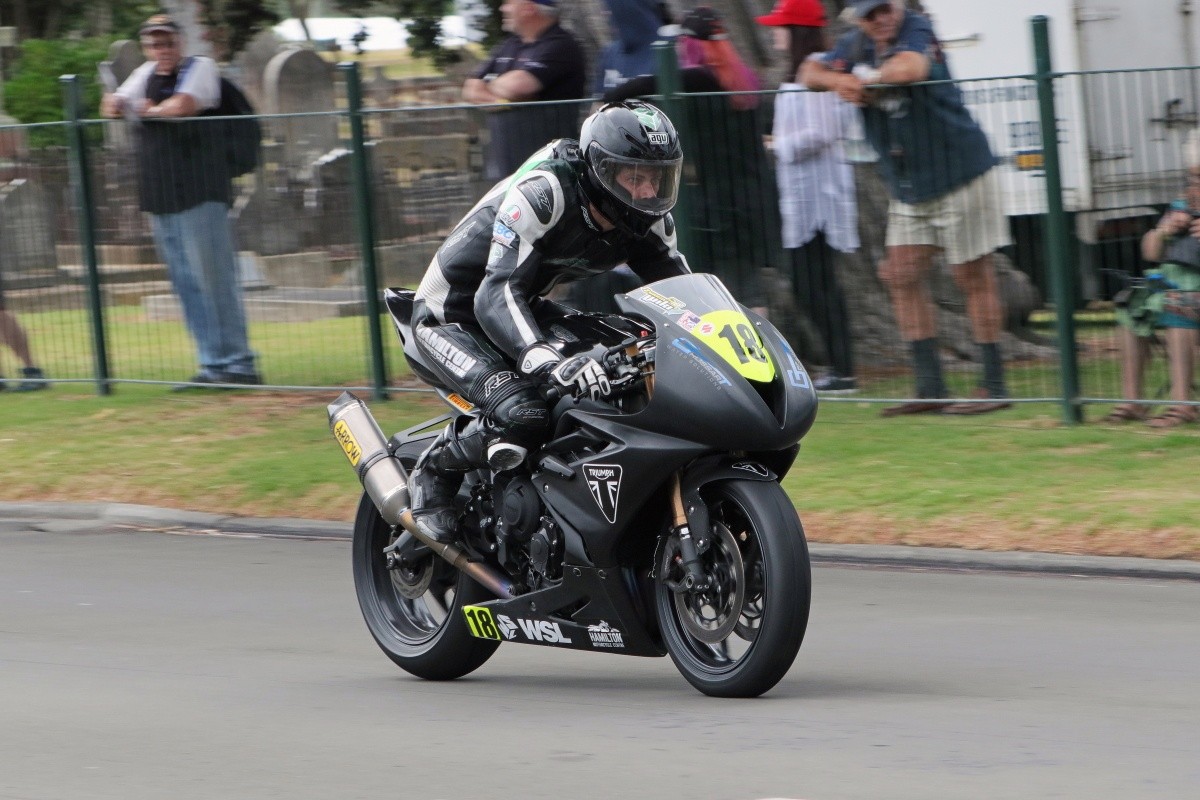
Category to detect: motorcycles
[324,268,819,702]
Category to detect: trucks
[910,0,1199,335]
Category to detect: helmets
[579,100,684,240]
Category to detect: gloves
[516,341,611,403]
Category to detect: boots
[406,415,488,541]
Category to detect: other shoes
[1102,405,1139,424]
[882,397,954,414]
[172,377,209,392]
[814,376,859,395]
[1149,404,1200,426]
[211,373,263,391]
[17,374,46,389]
[944,387,1012,413]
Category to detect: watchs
[482,72,499,84]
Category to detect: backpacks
[168,56,261,176]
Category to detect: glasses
[864,4,890,21]
[144,40,175,49]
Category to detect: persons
[458,0,1200,429]
[0,114,49,393]
[409,100,694,545]
[100,14,266,392]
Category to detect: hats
[534,0,555,6]
[756,0,828,27]
[849,0,889,16]
[137,14,180,35]
[657,7,728,41]
